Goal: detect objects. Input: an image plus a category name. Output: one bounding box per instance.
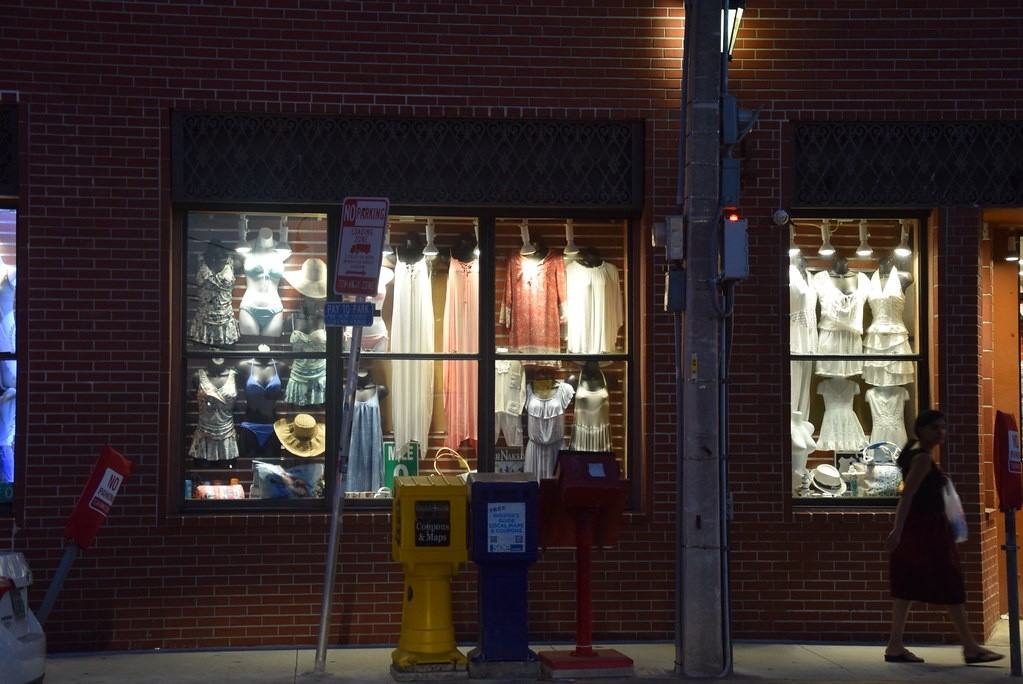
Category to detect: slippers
[964,649,1003,663]
[885,649,924,663]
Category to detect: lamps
[233,215,253,254]
[274,216,292,256]
[1003,249,1020,262]
[563,219,580,255]
[519,219,537,256]
[473,220,479,255]
[382,224,394,255]
[855,220,874,255]
[789,221,801,257]
[818,220,836,255]
[421,219,440,256]
[893,220,912,256]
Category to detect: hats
[809,464,847,495]
[273,413,325,457]
[282,258,327,298]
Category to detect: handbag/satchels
[942,477,968,543]
[433,447,478,495]
[847,441,905,497]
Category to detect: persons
[0,257,17,485]
[789,256,914,504]
[192,227,625,482]
[883,409,1004,663]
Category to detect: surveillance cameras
[774,210,789,225]
